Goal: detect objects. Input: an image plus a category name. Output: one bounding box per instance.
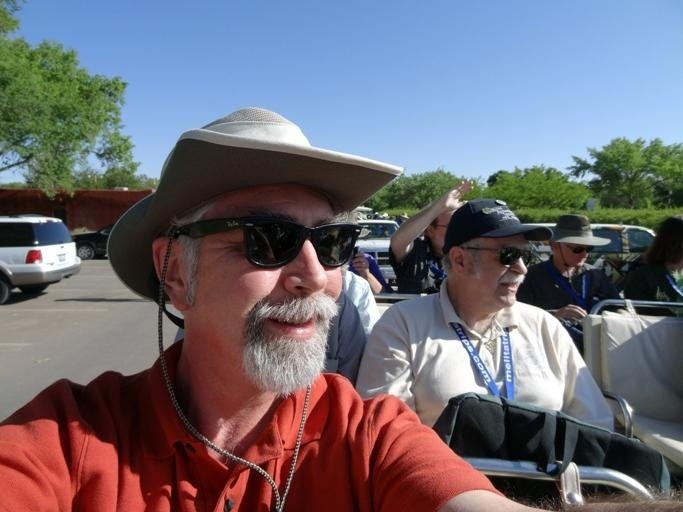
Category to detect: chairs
[373,295,681,510]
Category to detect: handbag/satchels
[429,392,674,509]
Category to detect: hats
[551,215,612,246]
[443,198,554,254]
[106,105,402,305]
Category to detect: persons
[170,269,380,389]
[386,177,473,294]
[515,210,627,354]
[354,195,616,437]
[0,106,682,511]
[621,214,682,315]
[371,211,409,238]
[344,251,388,293]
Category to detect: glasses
[459,245,532,267]
[172,213,363,269]
[566,243,595,254]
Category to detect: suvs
[0,214,85,305]
[351,220,402,280]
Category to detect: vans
[523,223,655,284]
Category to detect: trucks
[0,187,155,260]
